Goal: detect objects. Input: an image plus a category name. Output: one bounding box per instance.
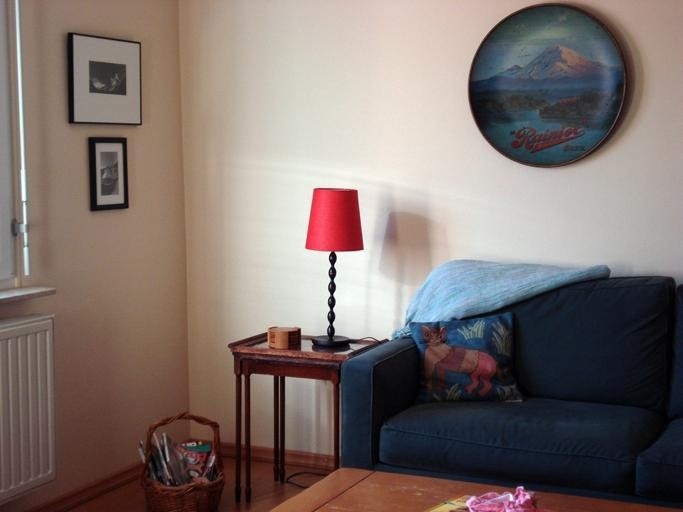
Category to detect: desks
[228,329,390,502]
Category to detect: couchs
[340,275,682,508]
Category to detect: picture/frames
[65,30,144,128]
[87,136,129,212]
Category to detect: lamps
[304,187,363,346]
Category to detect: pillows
[407,309,522,406]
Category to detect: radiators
[0,313,58,504]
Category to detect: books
[134,429,217,490]
[423,494,474,511]
